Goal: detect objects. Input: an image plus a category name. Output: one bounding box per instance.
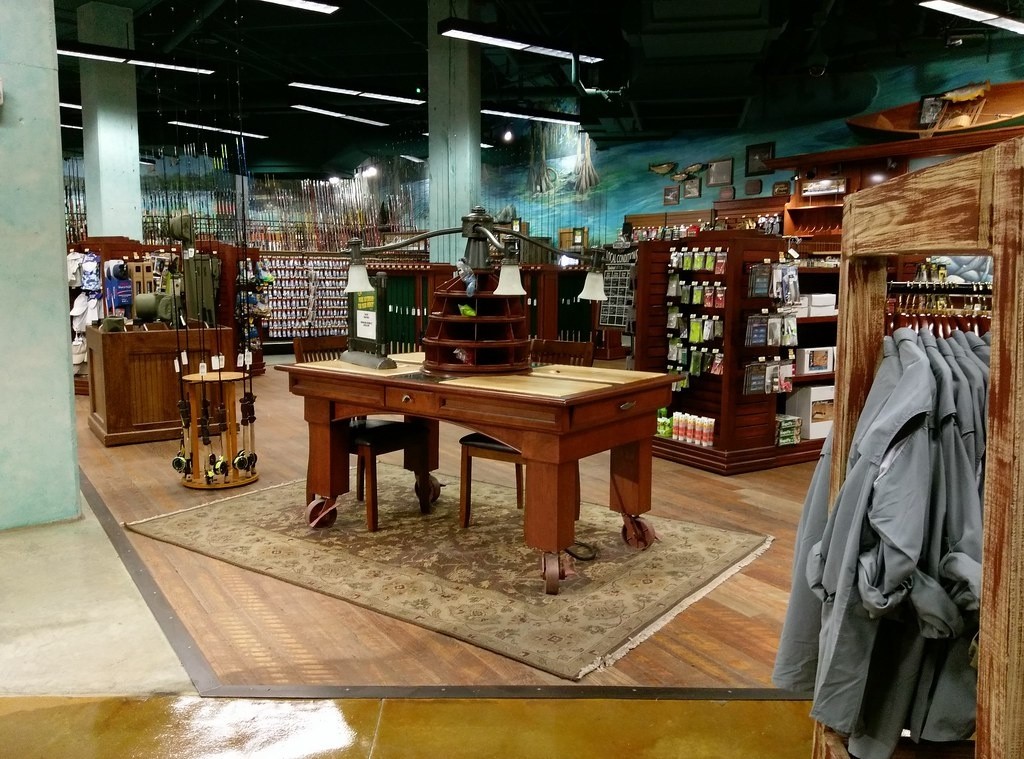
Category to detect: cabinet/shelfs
[84,318,233,446]
[180,370,259,488]
[421,269,533,376]
[596,204,843,476]
[65,236,184,395]
[196,241,267,376]
[258,249,431,355]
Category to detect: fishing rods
[158,142,259,480]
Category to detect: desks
[273,350,686,594]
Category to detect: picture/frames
[801,176,847,197]
[773,182,789,196]
[683,177,701,198]
[664,185,679,205]
[745,142,775,177]
[918,94,945,129]
[707,157,733,186]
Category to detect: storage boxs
[775,435,801,446]
[775,427,801,436]
[775,412,802,429]
[786,386,835,440]
[790,306,808,317]
[796,347,834,374]
[801,294,836,306]
[808,306,834,317]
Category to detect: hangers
[884,281,992,338]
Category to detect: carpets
[123,460,778,682]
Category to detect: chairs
[458,339,595,527]
[292,336,431,532]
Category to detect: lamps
[438,0,606,65]
[790,158,898,181]
[140,157,156,167]
[343,206,609,301]
[918,0,1024,35]
[480,101,580,126]
[56,22,216,75]
